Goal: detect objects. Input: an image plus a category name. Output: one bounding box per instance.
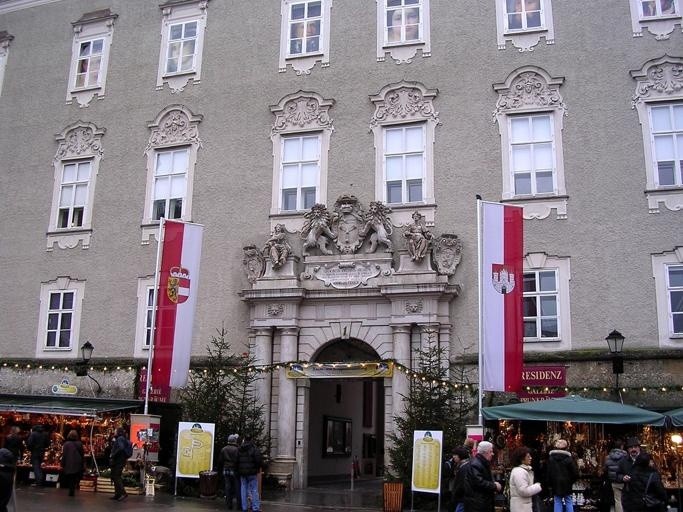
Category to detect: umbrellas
[479,394,667,427]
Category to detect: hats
[555,439,567,449]
[625,438,641,447]
[116,428,125,434]
[228,434,238,442]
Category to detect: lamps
[75,341,102,393]
[604,329,624,391]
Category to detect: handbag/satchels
[641,494,664,507]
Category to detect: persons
[263,223,291,268]
[442,437,663,512]
[218,433,241,511]
[402,210,430,261]
[236,434,264,511]
[0,422,132,512]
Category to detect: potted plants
[96,470,143,496]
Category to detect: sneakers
[118,493,127,501]
[109,494,122,500]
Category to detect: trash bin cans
[383,481,404,512]
[199,470,220,500]
